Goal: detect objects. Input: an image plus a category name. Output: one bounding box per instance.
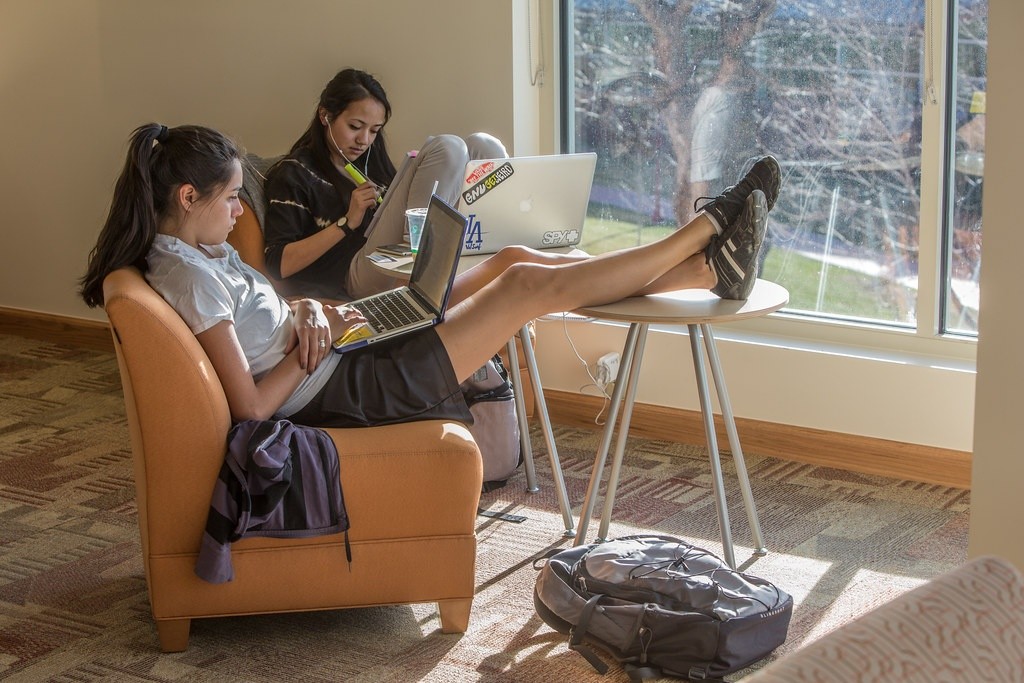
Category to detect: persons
[682,1,986,272]
[262,69,511,300]
[78,122,781,429]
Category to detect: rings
[317,339,325,348]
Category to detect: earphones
[324,114,330,125]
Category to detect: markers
[344,163,384,204]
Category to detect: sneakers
[695,156,781,233]
[706,189,769,300]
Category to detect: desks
[777,149,984,312]
[371,244,591,538]
[569,278,790,571]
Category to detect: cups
[405,208,428,262]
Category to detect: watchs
[337,216,353,237]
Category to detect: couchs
[103,265,483,653]
[226,153,537,424]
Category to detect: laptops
[330,194,468,354]
[458,152,598,256]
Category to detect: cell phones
[375,244,412,256]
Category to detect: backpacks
[534,535,792,682]
[454,355,523,494]
